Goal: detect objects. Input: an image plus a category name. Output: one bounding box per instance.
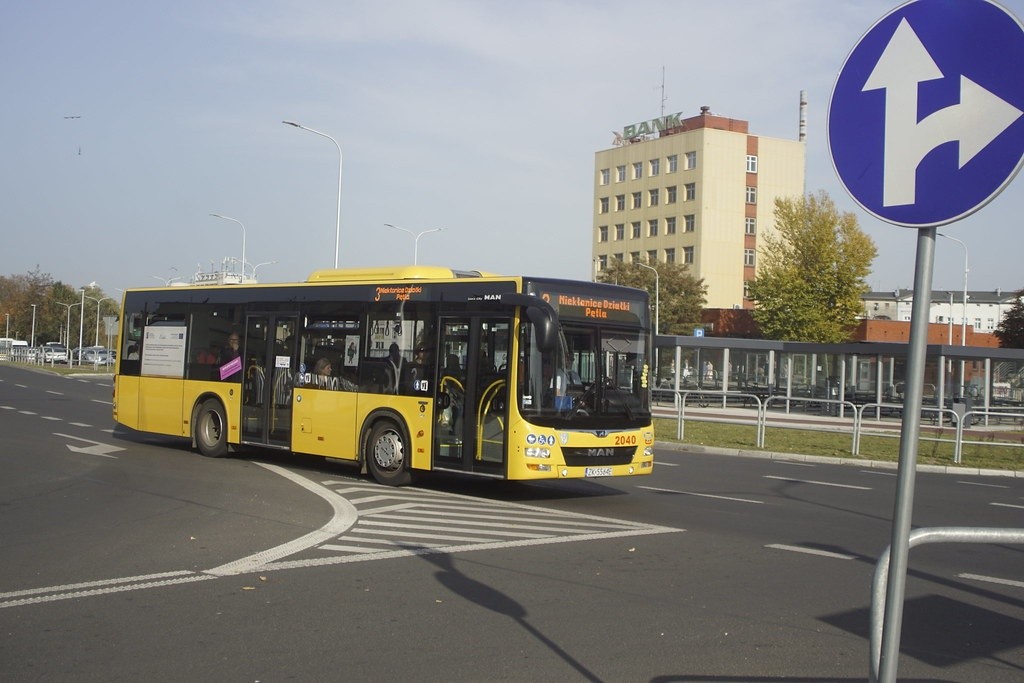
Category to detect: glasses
[566,358,575,361]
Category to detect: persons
[669,357,777,384]
[128,325,583,435]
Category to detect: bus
[111,266,655,487]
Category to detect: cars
[35,345,67,364]
[71,346,116,365]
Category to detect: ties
[568,371,574,385]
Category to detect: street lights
[31,304,36,349]
[232,257,279,279]
[84,296,112,346]
[54,302,81,355]
[207,213,246,284]
[5,314,10,348]
[935,232,968,397]
[383,223,446,266]
[78,289,86,365]
[281,120,343,269]
[636,262,659,375]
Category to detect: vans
[11,340,29,361]
